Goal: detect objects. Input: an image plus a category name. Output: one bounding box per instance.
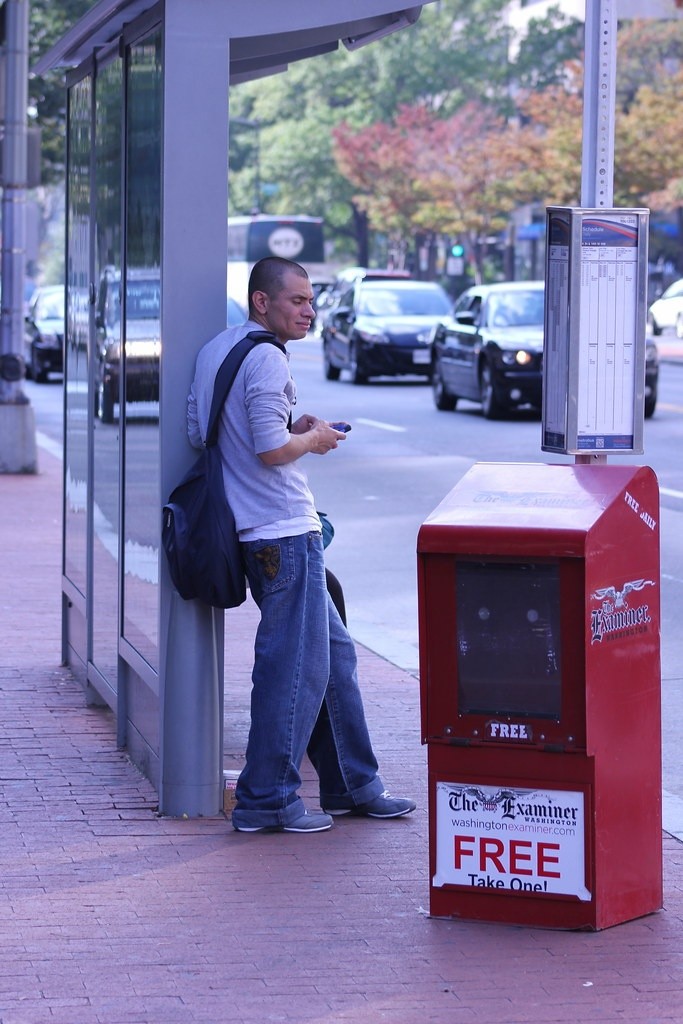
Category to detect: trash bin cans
[415,456,665,931]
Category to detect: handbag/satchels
[160,442,248,608]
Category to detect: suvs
[92,264,246,423]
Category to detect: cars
[22,283,66,384]
[312,267,414,339]
[648,275,682,340]
[319,278,457,388]
[428,277,664,423]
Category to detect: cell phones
[329,424,351,434]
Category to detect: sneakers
[322,790,416,818]
[235,809,333,832]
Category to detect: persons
[187,257,419,833]
